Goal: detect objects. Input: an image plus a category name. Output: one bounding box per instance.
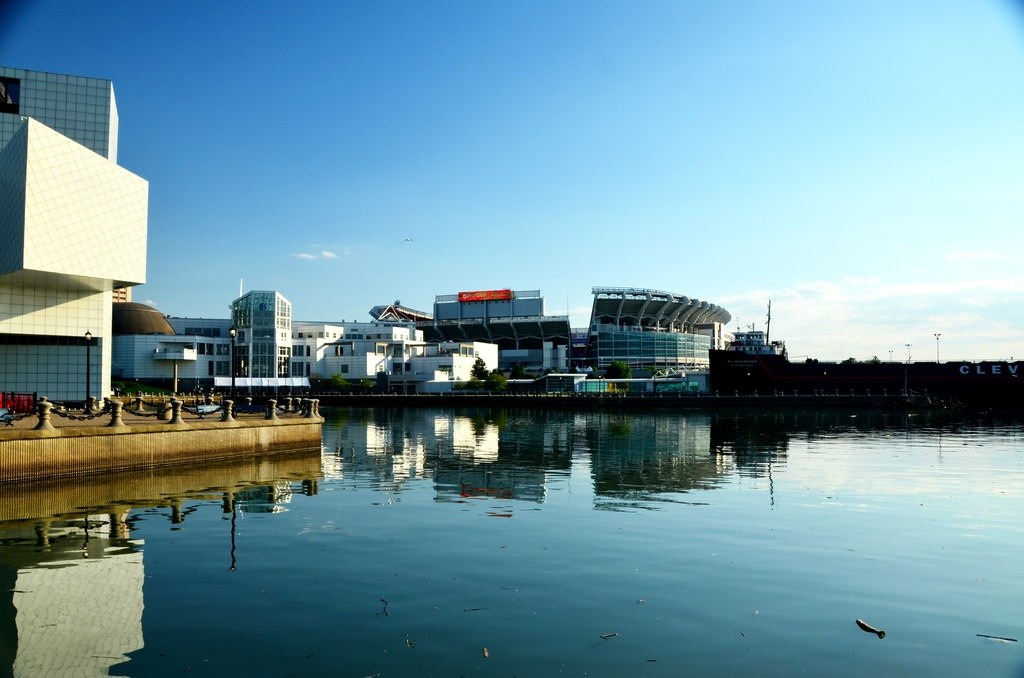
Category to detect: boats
[707,299,1024,409]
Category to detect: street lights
[84,327,92,415]
[229,325,238,418]
